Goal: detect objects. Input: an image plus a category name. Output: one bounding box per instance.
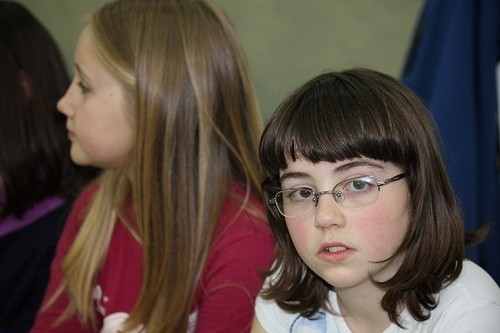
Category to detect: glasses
[267,170,409,218]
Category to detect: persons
[250,69,500,333]
[0,0,104,333]
[28,0,280,333]
